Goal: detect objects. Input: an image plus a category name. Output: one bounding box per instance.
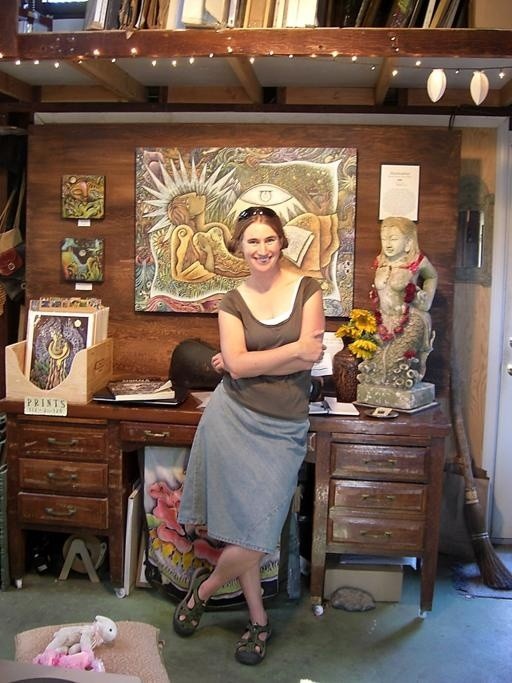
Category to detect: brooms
[448,375,512,591]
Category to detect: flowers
[335,307,379,361]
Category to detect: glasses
[238,208,276,219]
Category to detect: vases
[331,346,358,401]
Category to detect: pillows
[12,619,171,682]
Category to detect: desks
[0,380,452,610]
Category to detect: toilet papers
[62,533,106,574]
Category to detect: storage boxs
[5,334,113,405]
[321,557,406,605]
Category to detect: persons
[173,206,328,665]
[365,215,438,378]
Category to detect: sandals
[235,617,274,665]
[173,566,213,637]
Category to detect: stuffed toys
[34,614,118,673]
